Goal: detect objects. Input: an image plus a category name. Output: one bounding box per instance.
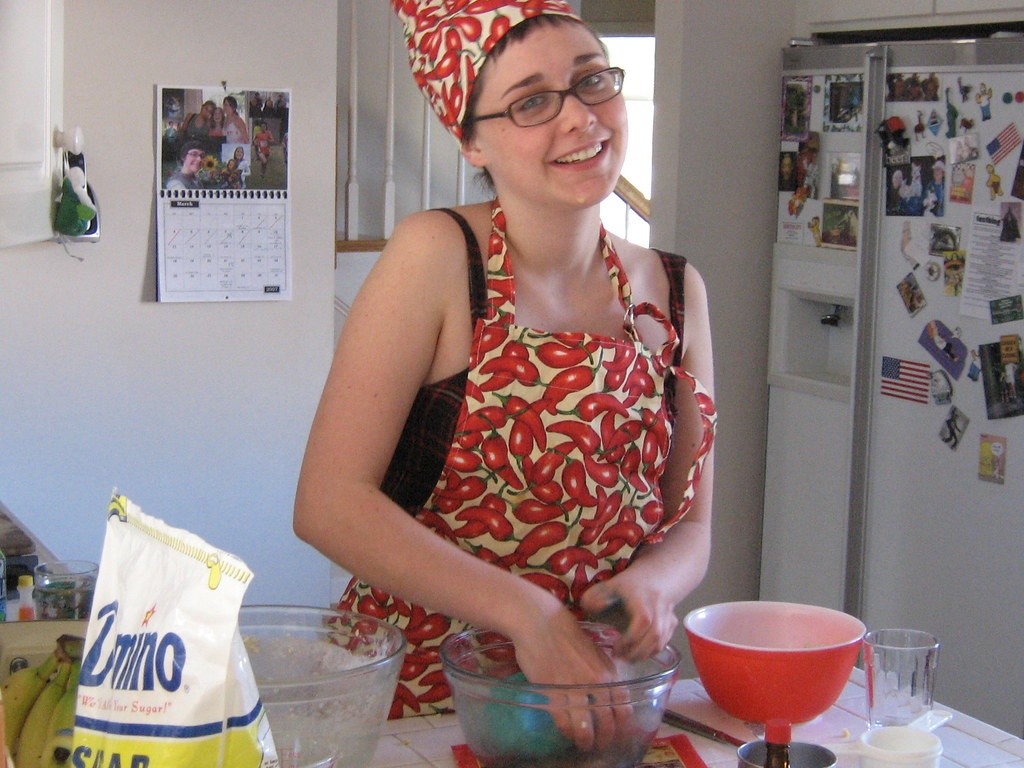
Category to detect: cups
[34,559,99,620]
[862,628,940,731]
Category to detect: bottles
[16,575,35,623]
[762,720,792,768]
[0,550,8,621]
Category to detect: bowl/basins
[440,618,682,767]
[736,739,838,768]
[683,599,867,724]
[238,603,407,768]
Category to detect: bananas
[0,634,87,768]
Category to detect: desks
[364,664,1024,768]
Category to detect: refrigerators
[762,30,1024,740]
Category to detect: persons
[289,0,717,748]
[891,160,944,215]
[999,362,1018,403]
[954,137,979,161]
[886,73,940,102]
[163,92,288,192]
[779,142,818,199]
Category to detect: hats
[390,0,582,147]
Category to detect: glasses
[466,66,629,128]
[187,152,205,158]
